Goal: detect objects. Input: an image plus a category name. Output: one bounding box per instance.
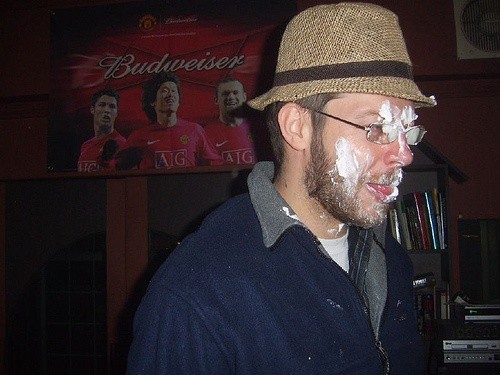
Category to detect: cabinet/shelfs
[388,163,452,319]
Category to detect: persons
[127,2,439,375]
[77,84,136,173]
[123,69,225,169]
[201,74,273,166]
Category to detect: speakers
[458,218,500,304]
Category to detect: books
[385,185,452,337]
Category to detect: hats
[247,3,437,113]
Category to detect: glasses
[296,100,427,146]
